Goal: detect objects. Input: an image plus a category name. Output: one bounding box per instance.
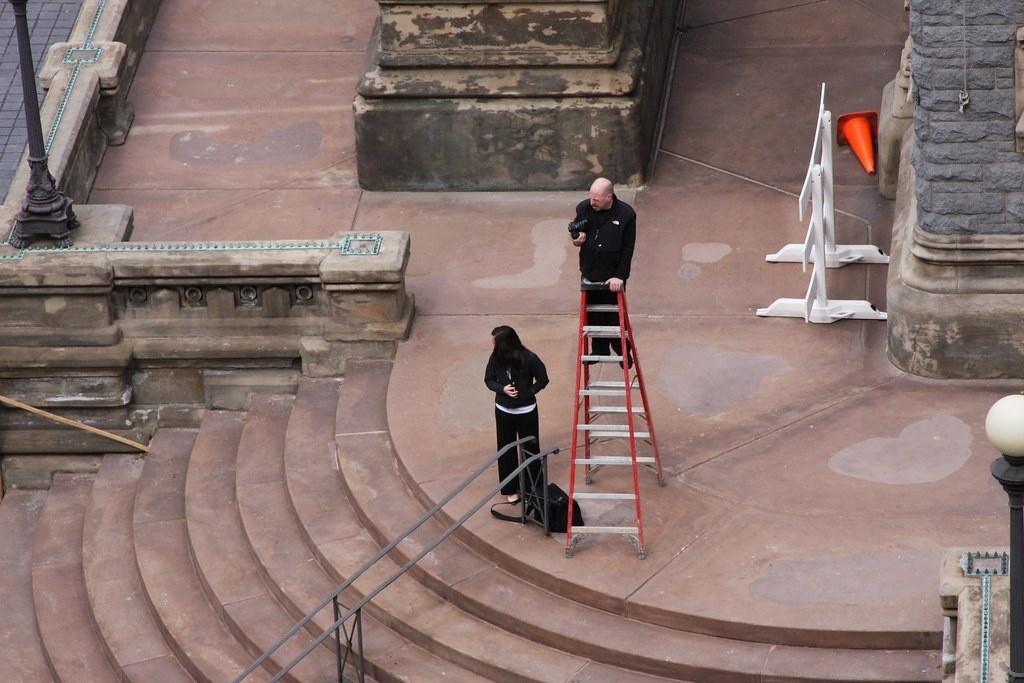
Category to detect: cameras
[568,219,590,238]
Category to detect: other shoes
[507,494,519,504]
[619,352,633,370]
[582,352,611,365]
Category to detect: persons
[572,177,636,369]
[485,325,549,504]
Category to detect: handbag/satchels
[527,483,584,533]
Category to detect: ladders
[563,282,666,559]
